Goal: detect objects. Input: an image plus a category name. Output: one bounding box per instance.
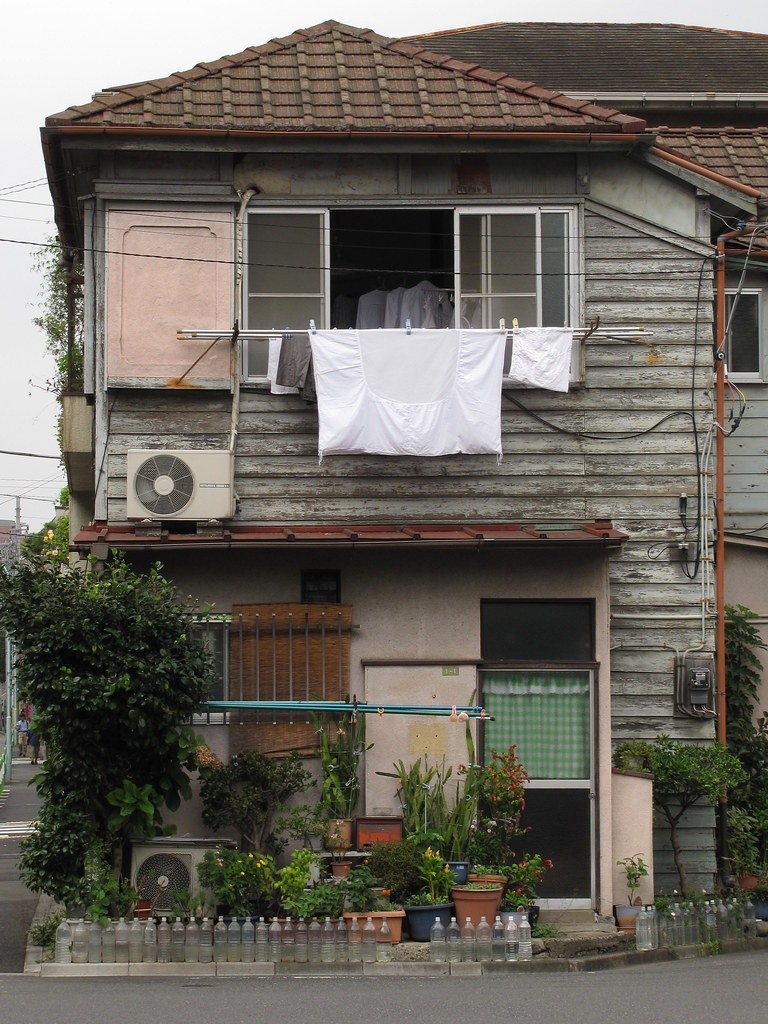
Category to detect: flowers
[501,889,531,911]
[320,819,351,863]
[508,853,554,906]
[471,743,532,864]
[308,710,364,818]
[407,846,460,906]
[196,847,275,914]
[338,860,400,912]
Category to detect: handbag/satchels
[27,739,30,745]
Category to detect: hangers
[420,272,430,283]
[420,286,479,304]
[378,272,391,291]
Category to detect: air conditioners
[130,845,222,918]
[126,448,236,521]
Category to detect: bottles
[157,918,172,963]
[242,917,255,962]
[143,918,157,962]
[172,917,185,962]
[55,917,73,963]
[88,919,101,963]
[129,918,143,963]
[199,917,213,962]
[185,917,199,963]
[429,915,533,963]
[635,897,756,951]
[116,917,130,963]
[101,918,116,963]
[228,917,241,962]
[255,916,392,963]
[73,918,89,962]
[214,916,228,962]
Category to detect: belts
[19,731,28,732]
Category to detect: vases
[325,819,354,853]
[501,905,540,938]
[223,915,258,945]
[342,904,406,944]
[331,861,350,879]
[404,902,454,941]
[497,911,529,930]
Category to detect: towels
[509,325,570,392]
[268,335,315,401]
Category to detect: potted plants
[451,882,503,928]
[394,752,476,886]
[273,803,324,851]
[468,864,510,898]
[615,853,649,933]
[263,848,339,943]
[726,806,761,890]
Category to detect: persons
[15,714,29,757]
[26,729,41,765]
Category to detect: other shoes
[35,761,37,764]
[31,761,34,765]
[19,753,22,756]
[23,755,25,757]
